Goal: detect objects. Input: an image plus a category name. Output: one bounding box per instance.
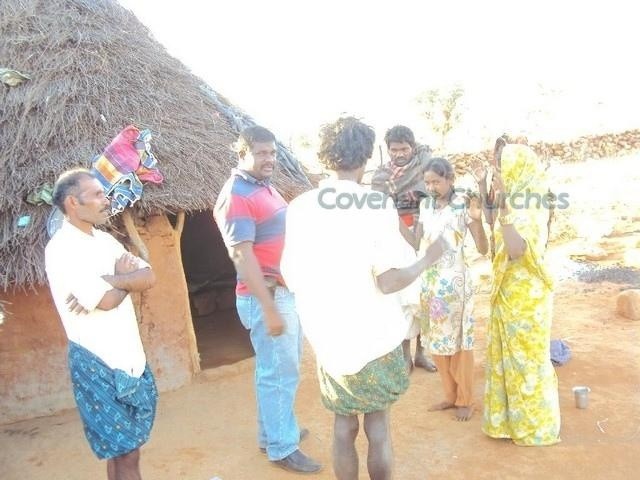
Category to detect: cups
[574,386,591,409]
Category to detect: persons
[213,124,325,475]
[42,169,159,480]
[398,158,489,423]
[278,115,451,480]
[370,125,438,377]
[465,129,563,448]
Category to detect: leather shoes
[270,449,323,474]
[261,429,308,453]
